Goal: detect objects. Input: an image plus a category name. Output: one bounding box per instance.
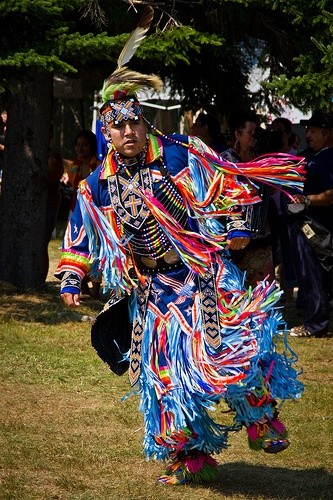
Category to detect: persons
[289,117,333,337]
[53,4,307,484]
[191,113,221,150]
[62,129,102,300]
[261,118,297,310]
[220,116,265,163]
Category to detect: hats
[300,110,333,129]
[99,67,165,126]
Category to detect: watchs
[303,195,311,207]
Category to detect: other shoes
[157,454,218,486]
[248,424,290,454]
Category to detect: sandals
[289,323,313,337]
